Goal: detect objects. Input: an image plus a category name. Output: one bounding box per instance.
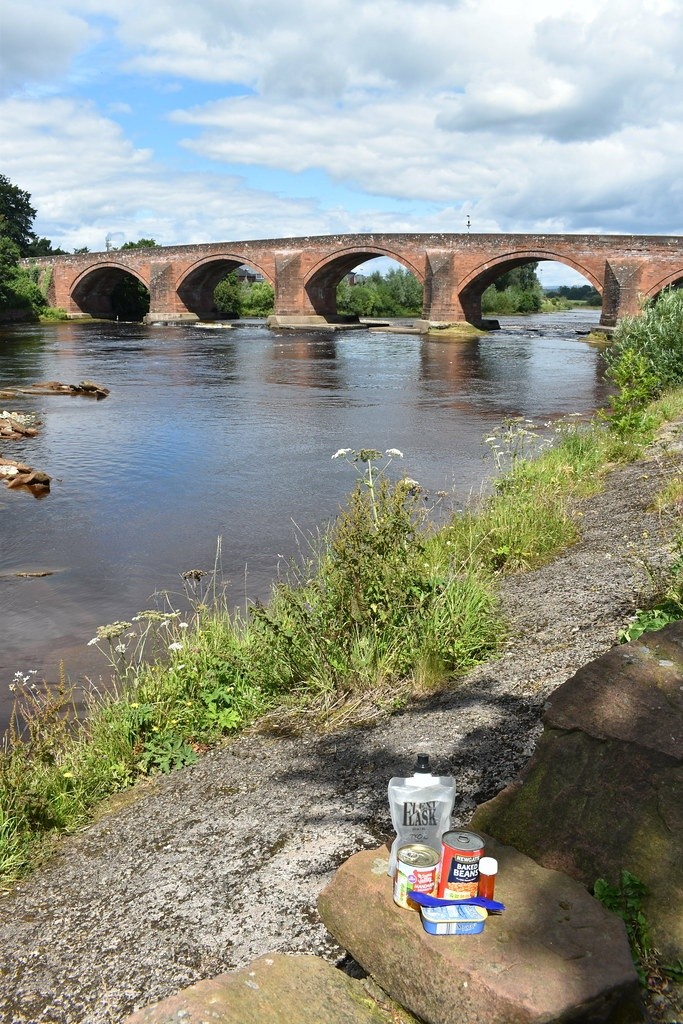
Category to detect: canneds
[437,830,486,899]
[394,843,443,911]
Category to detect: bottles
[478,856,498,901]
